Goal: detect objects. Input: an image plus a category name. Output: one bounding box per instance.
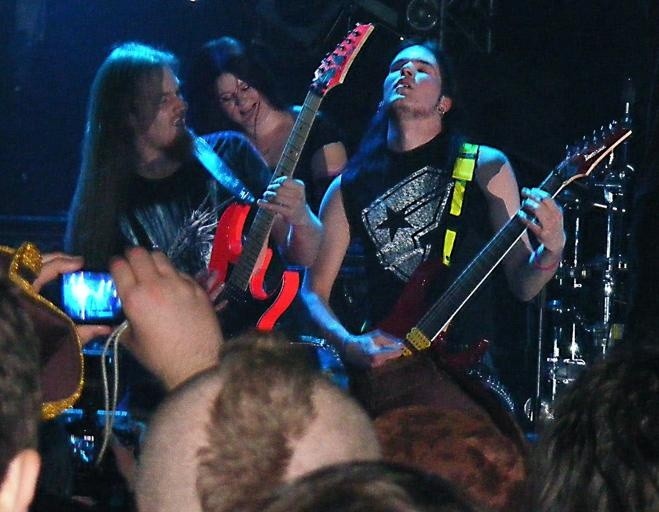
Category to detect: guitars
[196,22,374,335]
[363,120,631,383]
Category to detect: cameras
[59,269,125,326]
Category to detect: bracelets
[534,251,559,274]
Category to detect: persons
[293,36,568,389]
[524,321,659,512]
[265,458,486,512]
[61,40,325,354]
[181,32,348,218]
[137,327,384,512]
[0,240,225,512]
